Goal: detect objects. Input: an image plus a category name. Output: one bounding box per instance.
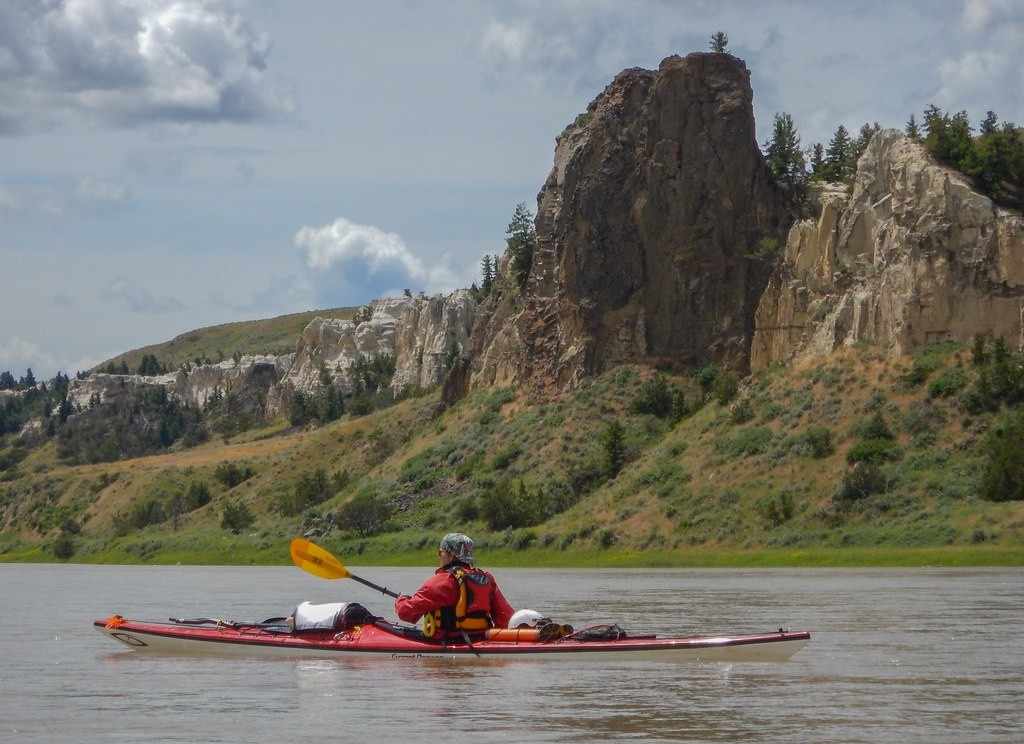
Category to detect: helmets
[508,609,545,629]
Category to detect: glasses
[438,550,447,557]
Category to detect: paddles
[168,616,295,635]
[289,539,400,598]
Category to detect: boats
[94,613,811,664]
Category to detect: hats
[441,533,475,563]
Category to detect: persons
[394,531,518,644]
